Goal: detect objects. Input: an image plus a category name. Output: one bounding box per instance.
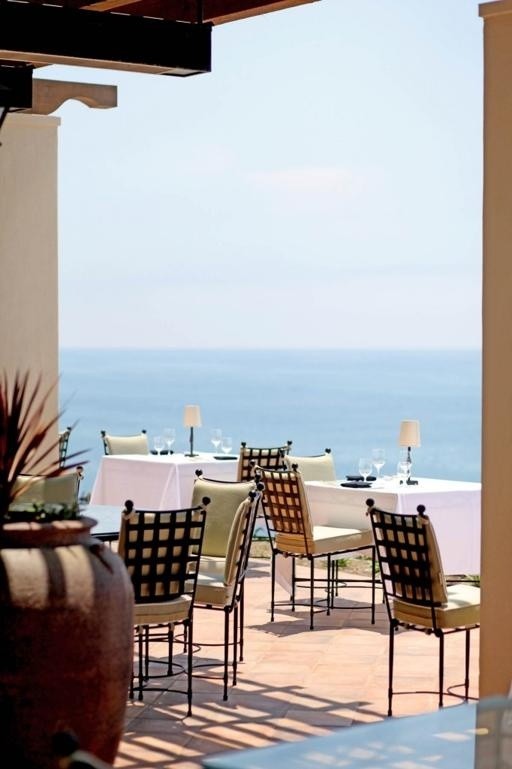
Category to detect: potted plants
[0,368,134,769]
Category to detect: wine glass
[372,453,386,479]
[359,458,372,482]
[153,436,166,457]
[211,428,222,454]
[163,427,175,456]
[397,462,412,489]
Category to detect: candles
[397,420,421,450]
[182,404,201,427]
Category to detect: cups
[221,437,233,456]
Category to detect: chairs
[367,499,481,715]
[118,469,266,715]
[101,430,149,456]
[11,464,84,520]
[237,440,293,482]
[282,448,336,481]
[250,460,377,631]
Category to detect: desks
[79,503,126,549]
